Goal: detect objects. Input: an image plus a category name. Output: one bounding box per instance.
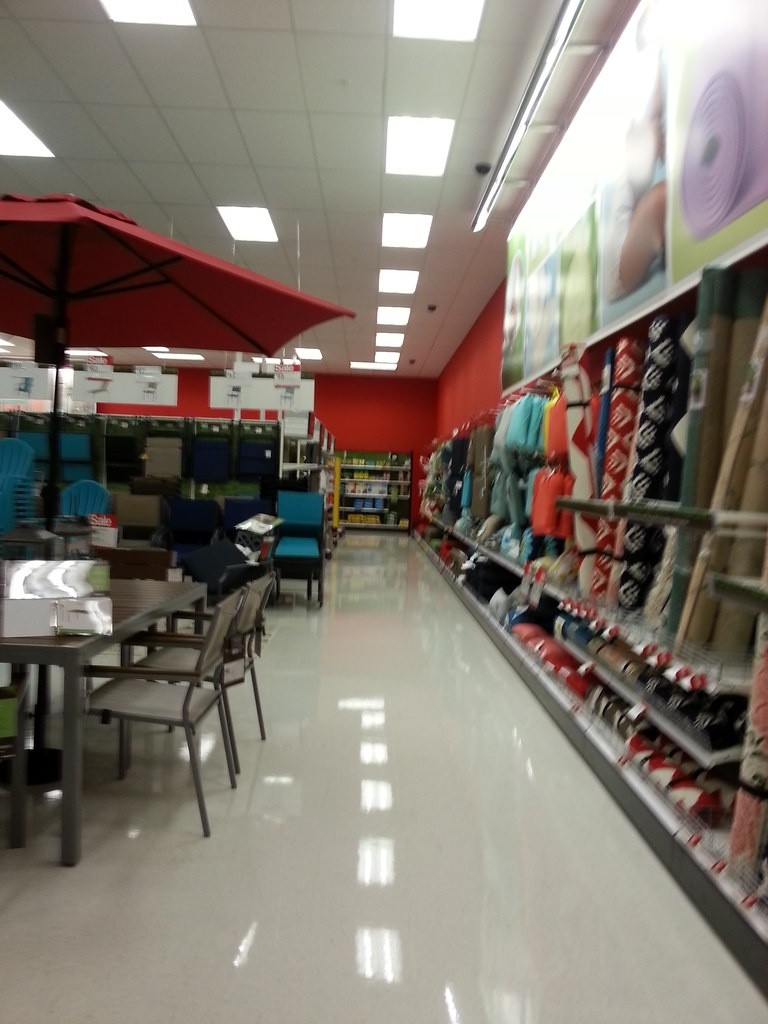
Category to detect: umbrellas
[1,188,359,566]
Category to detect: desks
[0,580,208,866]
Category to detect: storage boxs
[0,561,114,636]
[239,444,276,484]
[193,438,231,483]
[106,436,142,484]
[146,436,183,484]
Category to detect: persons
[603,72,669,309]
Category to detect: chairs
[58,478,111,517]
[82,585,248,838]
[76,547,178,724]
[115,491,327,608]
[1,436,40,533]
[135,571,277,741]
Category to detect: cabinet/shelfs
[412,229,768,1008]
[97,415,412,559]
